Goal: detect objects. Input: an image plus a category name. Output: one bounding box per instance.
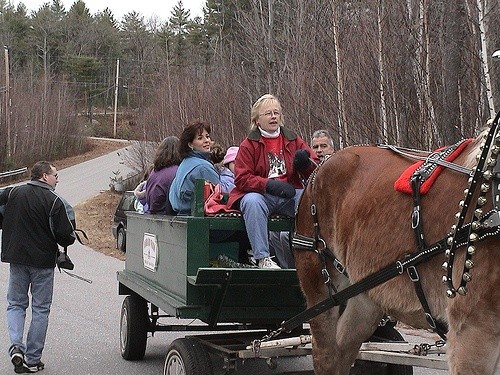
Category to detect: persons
[227,93,320,271]
[309,129,335,161]
[1,159,75,373]
[134,118,239,217]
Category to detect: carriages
[115,110,500,375]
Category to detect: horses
[293,125,500,375]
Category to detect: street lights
[114,58,128,138]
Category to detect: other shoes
[258,257,281,270]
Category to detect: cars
[111,191,137,251]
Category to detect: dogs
[0,187,76,258]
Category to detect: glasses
[45,172,58,180]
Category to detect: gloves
[267,178,298,198]
[293,148,312,170]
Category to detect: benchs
[192,181,294,232]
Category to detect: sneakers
[25,362,45,373]
[11,348,30,375]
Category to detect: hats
[223,146,239,164]
